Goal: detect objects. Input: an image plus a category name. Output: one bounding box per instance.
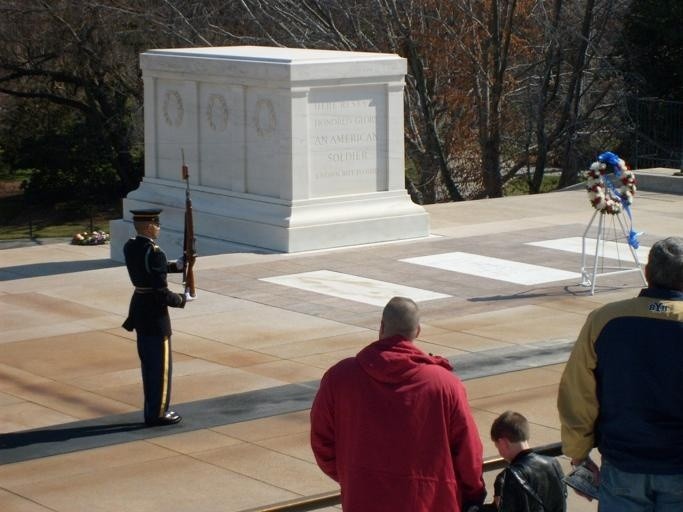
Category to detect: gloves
[185,292,198,302]
[176,255,185,270]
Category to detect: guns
[181,150,195,300]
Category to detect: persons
[556,235,682,512]
[308,295,488,511]
[120,205,184,425]
[489,410,570,512]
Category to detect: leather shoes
[144,410,182,426]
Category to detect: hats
[129,208,163,224]
